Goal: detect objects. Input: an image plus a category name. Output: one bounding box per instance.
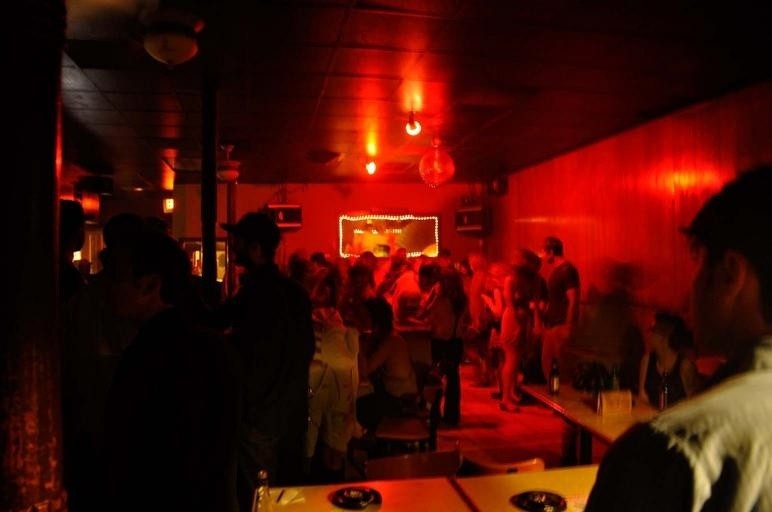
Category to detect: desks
[522,387,651,464]
[253,473,474,512]
[453,465,600,511]
[577,410,661,463]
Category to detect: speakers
[269,205,302,229]
[456,208,483,232]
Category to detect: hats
[221,211,279,249]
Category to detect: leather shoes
[492,390,525,412]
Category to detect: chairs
[369,373,447,460]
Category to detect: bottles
[256,470,273,511]
[549,357,560,395]
[658,367,673,411]
[592,361,608,414]
[610,364,622,390]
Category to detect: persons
[586,162,772,512]
[61,199,579,512]
[637,311,702,413]
